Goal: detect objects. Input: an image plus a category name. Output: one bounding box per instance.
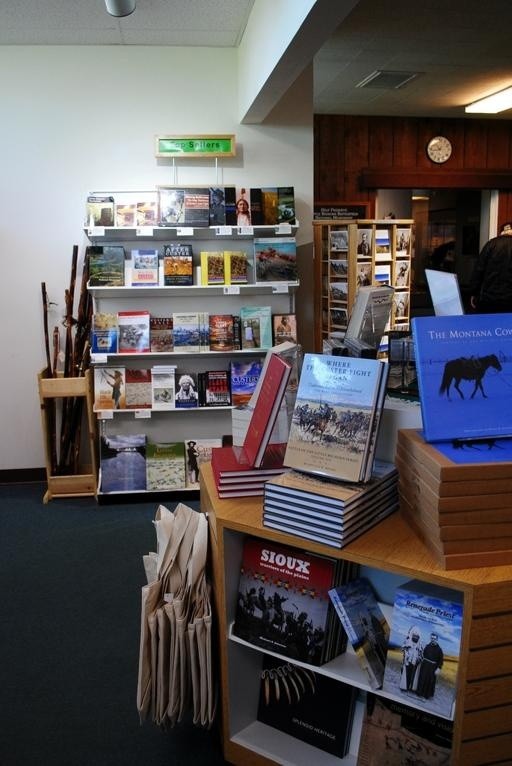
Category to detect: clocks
[426,136,452,164]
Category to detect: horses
[437,353,503,400]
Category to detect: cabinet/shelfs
[196,460,511,766]
[311,219,415,360]
[83,184,299,507]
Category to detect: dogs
[176,374,196,400]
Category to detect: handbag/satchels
[136,551,214,734]
